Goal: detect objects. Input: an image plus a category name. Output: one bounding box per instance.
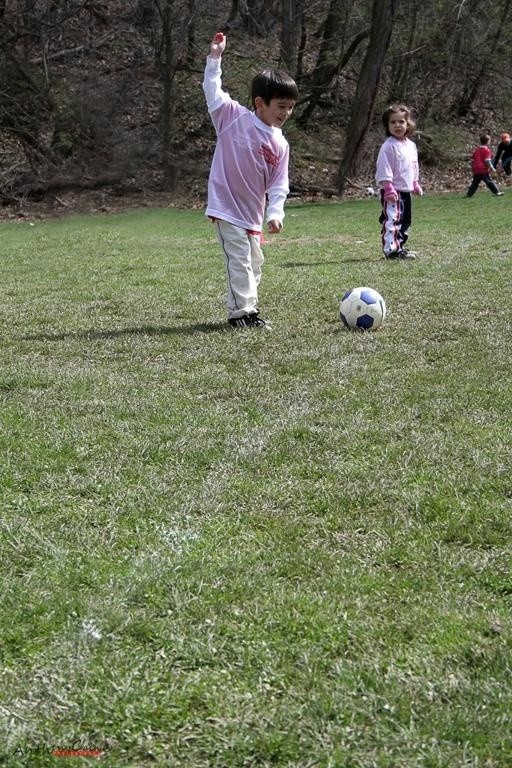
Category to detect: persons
[493,132,512,178]
[375,104,424,260]
[464,134,506,198]
[202,32,300,330]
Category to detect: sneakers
[227,312,273,332]
[496,191,504,196]
[399,248,418,260]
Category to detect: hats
[500,133,510,142]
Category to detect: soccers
[340,287,386,332]
